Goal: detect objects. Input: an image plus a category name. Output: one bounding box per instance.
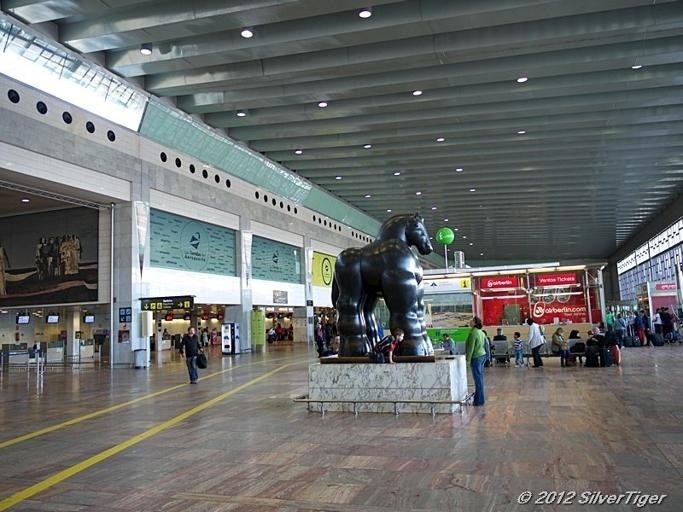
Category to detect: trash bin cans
[134,349,147,369]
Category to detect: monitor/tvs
[83,315,95,323]
[16,315,30,324]
[46,315,59,324]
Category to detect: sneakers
[190,381,197,384]
[514,361,543,368]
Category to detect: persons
[551,306,676,368]
[494,327,507,363]
[177,326,205,384]
[311,324,339,357]
[266,322,293,344]
[365,327,405,364]
[526,318,547,369]
[439,333,457,354]
[464,316,491,405]
[197,326,218,346]
[513,332,525,368]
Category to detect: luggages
[611,345,621,366]
[624,327,641,347]
[648,330,665,346]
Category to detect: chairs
[453,339,600,367]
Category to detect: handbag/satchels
[197,354,207,369]
[539,326,545,335]
